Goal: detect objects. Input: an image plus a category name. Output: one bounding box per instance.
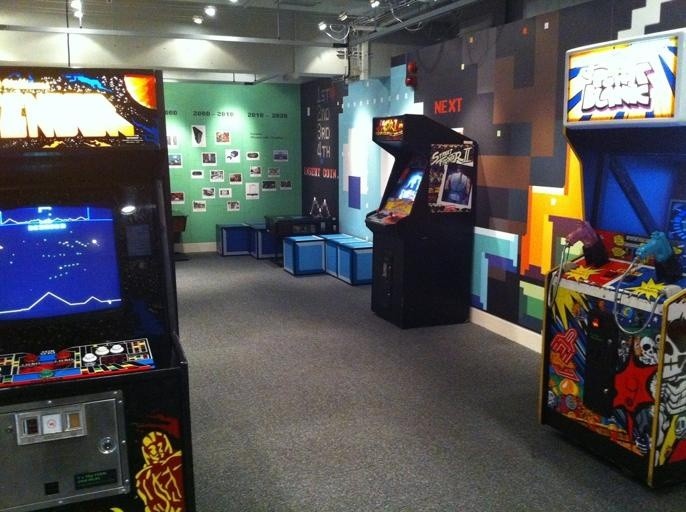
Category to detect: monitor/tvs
[0,203,124,323]
[591,152,686,240]
[396,169,425,202]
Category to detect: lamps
[72,1,83,28]
[204,3,216,17]
[370,0,380,9]
[193,15,204,25]
[318,21,327,30]
[336,10,347,22]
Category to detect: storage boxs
[216,215,374,286]
